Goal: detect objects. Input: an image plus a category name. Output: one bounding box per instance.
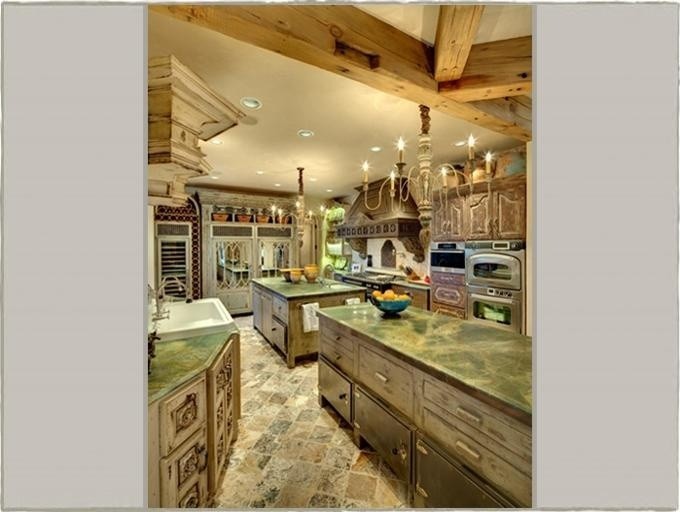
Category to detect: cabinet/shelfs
[147,332,241,508]
[390,281,429,308]
[430,180,526,336]
[146,193,200,303]
[316,301,532,508]
[201,203,302,315]
[251,278,368,369]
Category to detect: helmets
[371,299,411,319]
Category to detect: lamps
[269,166,329,235]
[359,103,493,213]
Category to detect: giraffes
[342,272,404,289]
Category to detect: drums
[403,266,413,275]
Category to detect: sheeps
[147,273,194,321]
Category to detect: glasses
[269,166,329,235]
[359,103,493,213]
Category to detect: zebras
[430,240,526,336]
